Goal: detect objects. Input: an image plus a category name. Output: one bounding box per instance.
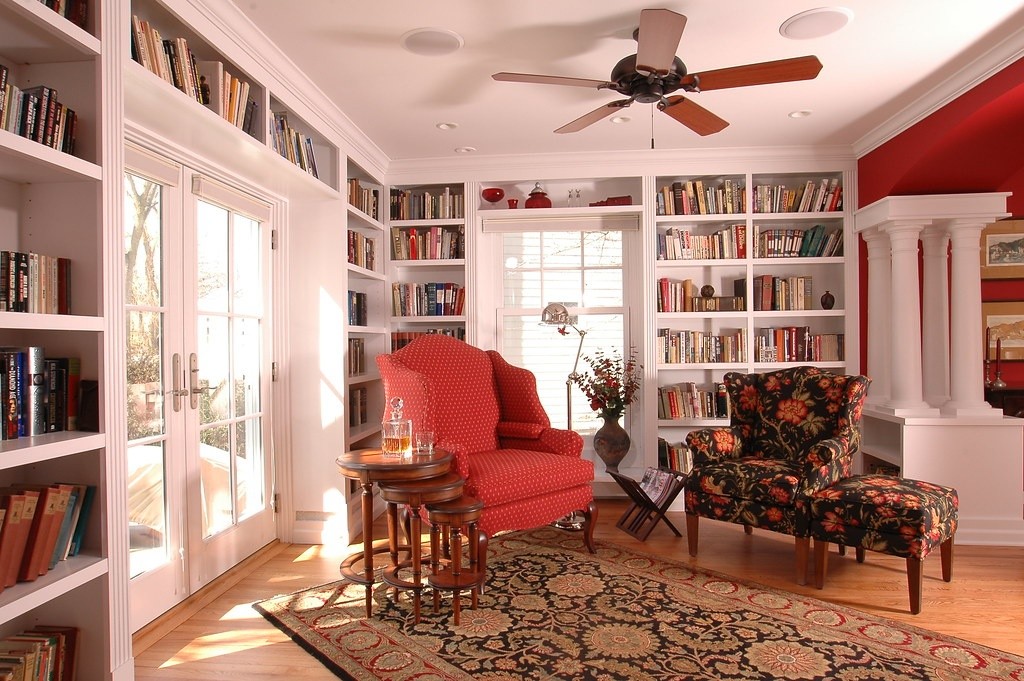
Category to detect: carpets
[250,525,1024,681]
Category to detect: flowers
[571,346,645,418]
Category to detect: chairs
[374,333,597,598]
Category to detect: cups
[415,431,435,456]
[507,199,518,209]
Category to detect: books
[347,289,369,327]
[350,448,375,493]
[654,172,850,366]
[390,280,466,317]
[0,482,98,594]
[269,106,320,179]
[633,434,701,505]
[346,335,367,378]
[389,224,466,261]
[33,0,91,30]
[348,387,368,427]
[865,463,900,479]
[0,342,99,448]
[390,185,467,220]
[0,625,82,681]
[0,62,78,158]
[346,172,380,272]
[657,381,731,419]
[0,250,73,315]
[389,326,467,355]
[131,13,259,137]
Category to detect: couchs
[683,364,872,586]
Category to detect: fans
[491,28,823,133]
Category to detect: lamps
[542,303,588,530]
[635,9,687,78]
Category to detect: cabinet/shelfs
[1,0,856,681]
[857,407,1024,544]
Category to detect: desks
[334,446,464,626]
[985,387,1024,418]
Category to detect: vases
[593,415,630,472]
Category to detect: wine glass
[482,188,505,209]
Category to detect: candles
[985,326,991,360]
[997,338,1000,372]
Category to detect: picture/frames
[981,298,1024,361]
[981,218,1024,280]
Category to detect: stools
[423,497,484,625]
[811,475,959,613]
[606,466,690,543]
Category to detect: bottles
[381,396,413,458]
[567,189,573,207]
[821,290,835,310]
[575,189,581,206]
[524,182,552,208]
